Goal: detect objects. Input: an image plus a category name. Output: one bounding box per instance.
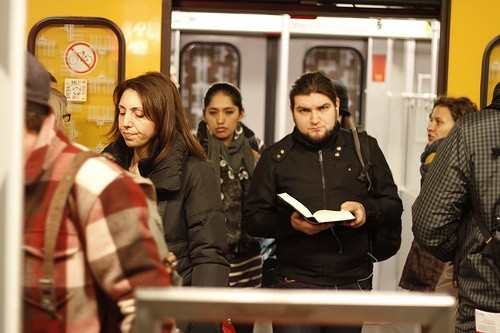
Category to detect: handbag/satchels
[357,131,402,261]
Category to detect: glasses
[63,114,71,122]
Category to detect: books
[276,192,356,225]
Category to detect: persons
[100,71,229,333]
[411,82,500,333]
[242,71,403,333]
[397,96,479,333]
[195,82,264,333]
[22,53,175,333]
[331,79,356,132]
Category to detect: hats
[26,53,52,109]
[331,80,351,117]
